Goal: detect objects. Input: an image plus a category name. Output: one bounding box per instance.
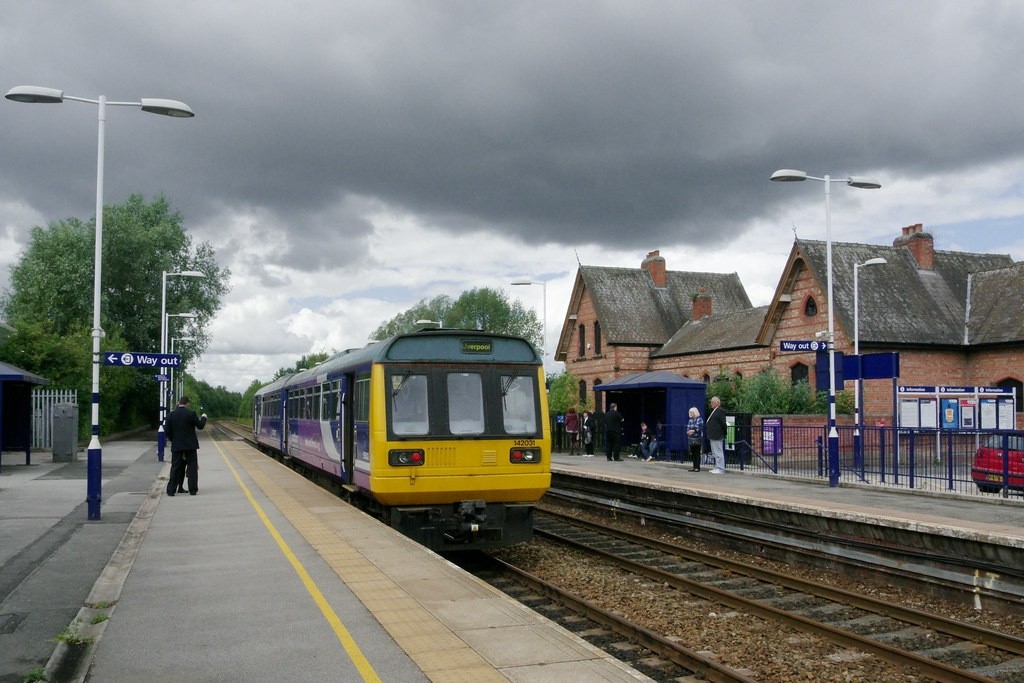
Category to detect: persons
[564,406,581,455]
[604,402,624,461]
[628,422,654,458]
[687,407,704,472]
[164,396,208,496]
[705,395,727,474]
[581,408,596,457]
[642,421,665,461]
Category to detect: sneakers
[712,469,725,475]
[709,468,716,473]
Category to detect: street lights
[768,168,883,484]
[509,280,548,384]
[4,83,197,524]
[156,270,206,465]
[414,318,444,329]
[851,257,888,472]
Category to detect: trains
[252,326,552,566]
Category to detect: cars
[971,432,1024,494]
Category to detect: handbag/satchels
[689,436,702,447]
[584,427,592,445]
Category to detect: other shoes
[642,458,645,461]
[178,487,187,493]
[576,451,581,456]
[588,455,594,458]
[688,468,695,471]
[569,452,574,456]
[582,455,587,457]
[694,468,700,472]
[646,456,653,462]
[627,454,632,458]
[614,459,624,461]
[632,455,638,459]
[607,458,612,461]
